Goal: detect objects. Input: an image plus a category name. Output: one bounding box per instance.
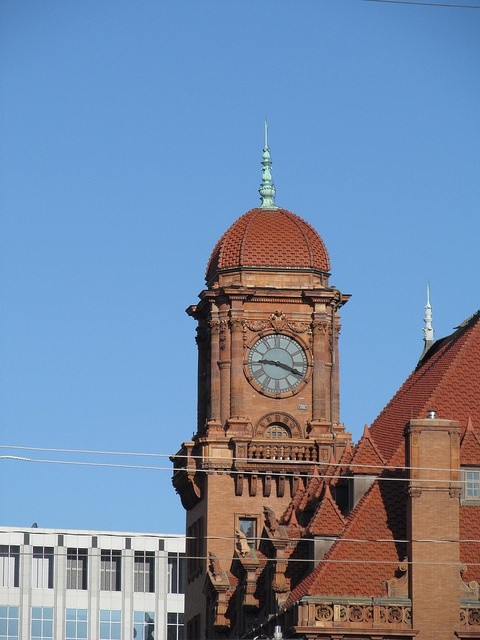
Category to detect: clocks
[243,328,315,401]
